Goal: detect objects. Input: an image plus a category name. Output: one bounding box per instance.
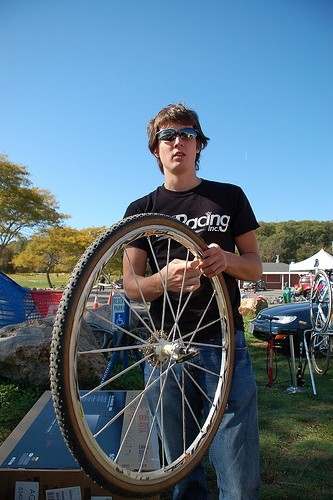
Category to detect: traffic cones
[107,291,112,304]
[92,295,98,309]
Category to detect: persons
[123,104,262,500]
[99,275,105,291]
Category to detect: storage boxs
[0,386,188,500]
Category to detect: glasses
[156,127,199,141]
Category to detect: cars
[298,276,317,301]
[252,298,329,342]
[243,278,267,292]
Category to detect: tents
[289,248,333,288]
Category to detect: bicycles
[303,268,333,395]
